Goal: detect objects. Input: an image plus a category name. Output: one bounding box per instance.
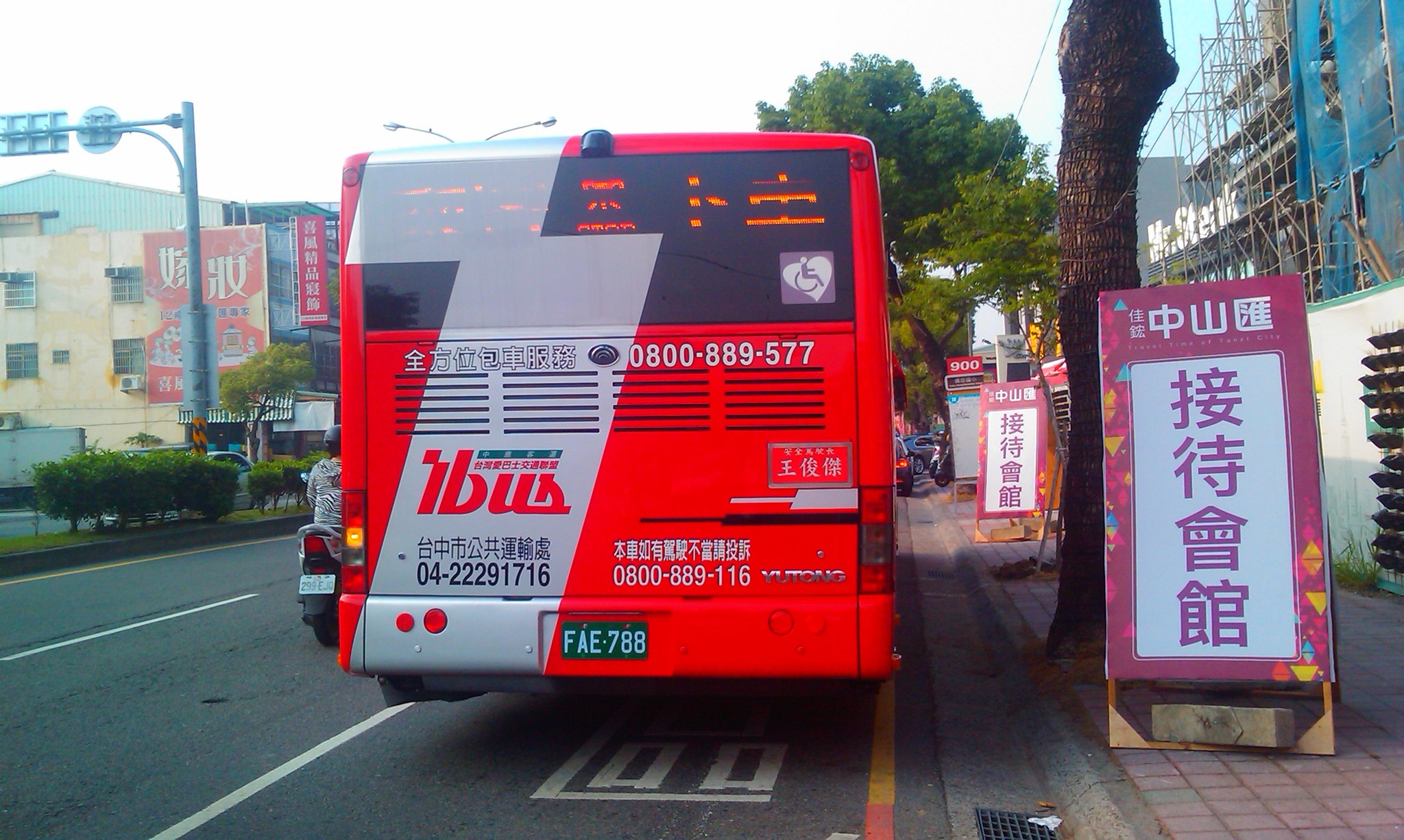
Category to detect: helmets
[323,425,341,447]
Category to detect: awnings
[176,390,295,424]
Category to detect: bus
[335,129,911,702]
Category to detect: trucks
[0,426,89,506]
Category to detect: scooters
[929,443,956,485]
[295,471,342,641]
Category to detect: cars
[893,428,917,495]
[903,433,950,467]
[204,451,273,495]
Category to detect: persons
[306,425,342,534]
